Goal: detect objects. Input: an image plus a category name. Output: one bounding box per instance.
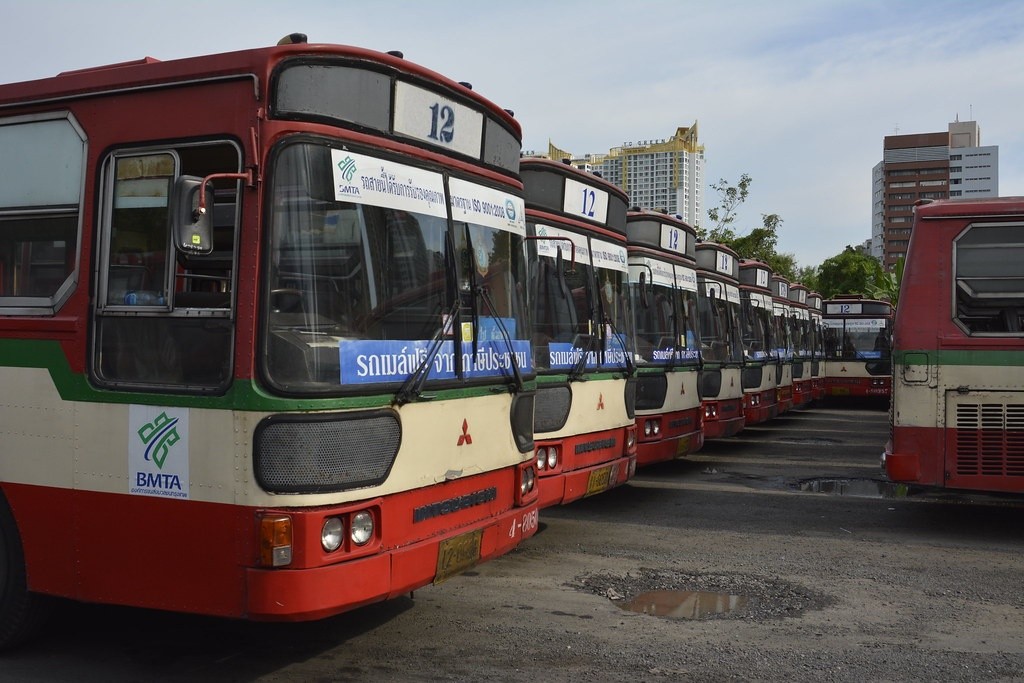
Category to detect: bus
[695,241,746,438]
[739,258,780,425]
[770,276,794,415]
[809,292,827,399]
[626,204,706,468]
[518,156,637,508]
[882,197,1024,506]
[789,283,813,405]
[821,292,896,399]
[0,29,539,626]
